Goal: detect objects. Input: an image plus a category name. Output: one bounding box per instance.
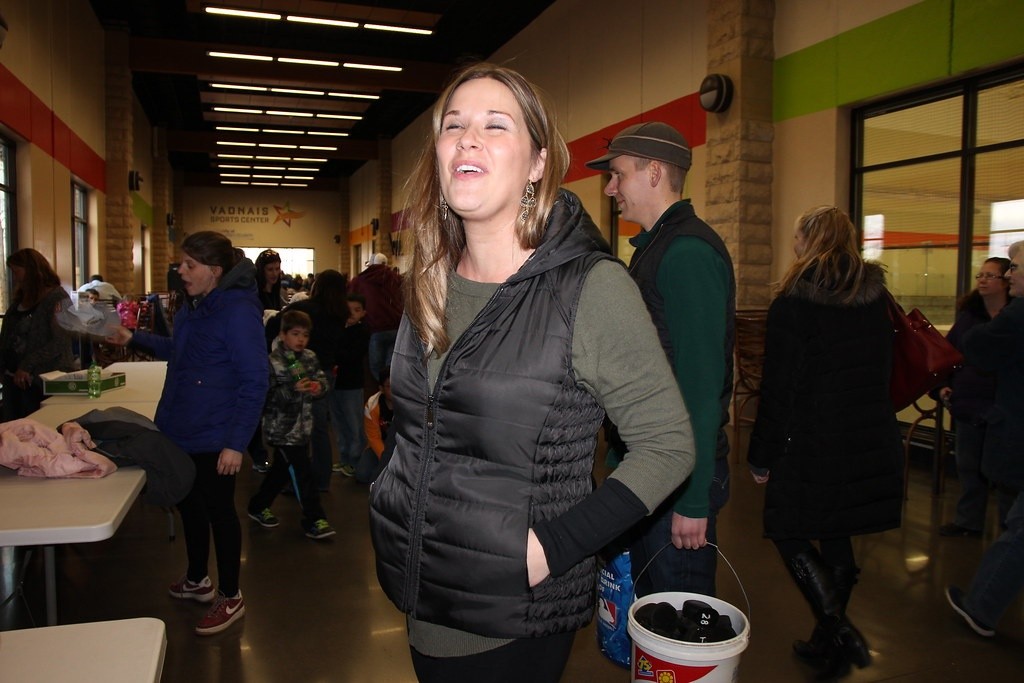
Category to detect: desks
[35,360,168,402]
[0,616,167,683]
[0,402,165,628]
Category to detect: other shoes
[340,465,353,476]
[252,464,268,472]
[938,523,983,537]
[332,462,345,471]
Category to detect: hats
[585,122,691,170]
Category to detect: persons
[246,311,337,538]
[0,248,78,424]
[247,249,289,471]
[104,231,268,635]
[367,63,695,682]
[359,366,392,478]
[945,241,1024,636]
[281,270,320,308]
[350,254,403,381]
[587,121,736,600]
[274,269,350,412]
[76,275,122,370]
[746,205,906,682]
[932,258,1014,538]
[332,296,372,477]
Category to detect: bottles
[286,354,311,389]
[88,360,102,400]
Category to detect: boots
[785,547,871,679]
[793,562,859,662]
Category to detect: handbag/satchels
[881,287,964,414]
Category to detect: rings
[759,477,761,480]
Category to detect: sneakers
[305,519,336,539]
[195,589,245,634]
[168,576,216,602]
[248,506,280,527]
[944,583,995,636]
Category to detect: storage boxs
[37,372,126,396]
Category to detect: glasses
[975,274,1003,279]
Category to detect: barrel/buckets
[627,542,751,682]
